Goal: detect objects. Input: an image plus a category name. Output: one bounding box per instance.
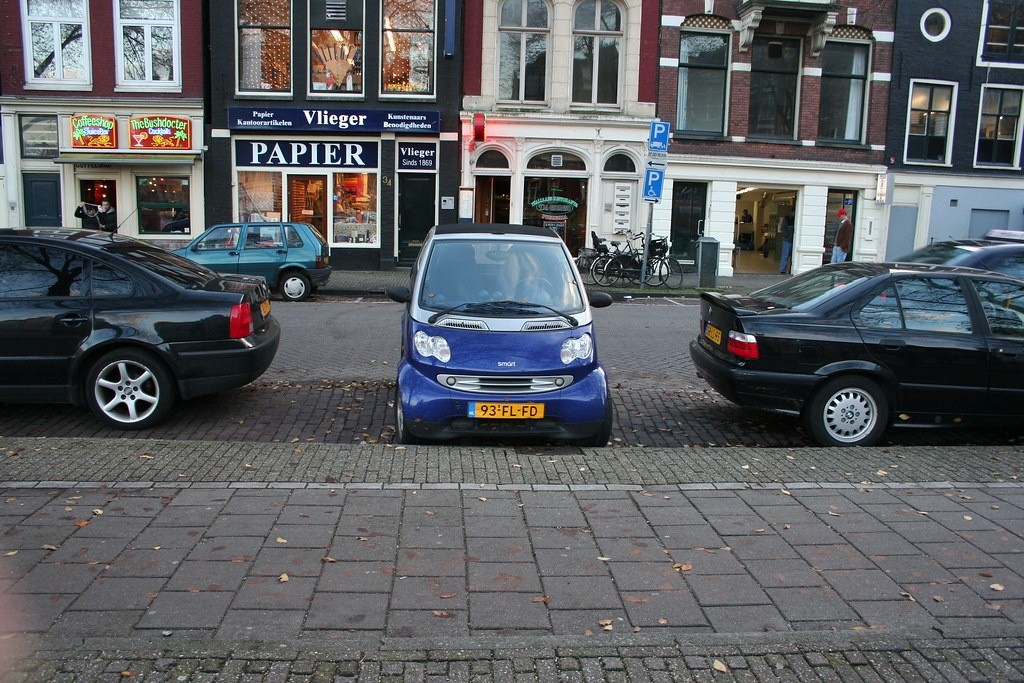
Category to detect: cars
[0,227,282,430]
[163,218,191,232]
[837,230,1024,312]
[387,222,613,447]
[170,222,331,301]
[688,261,1023,446]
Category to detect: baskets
[648,239,668,256]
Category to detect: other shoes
[788,272,791,275]
[781,272,785,274]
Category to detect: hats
[835,209,848,217]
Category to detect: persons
[311,189,323,234]
[741,210,753,244]
[831,209,853,264]
[778,207,795,274]
[73,200,117,233]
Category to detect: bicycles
[575,229,684,289]
